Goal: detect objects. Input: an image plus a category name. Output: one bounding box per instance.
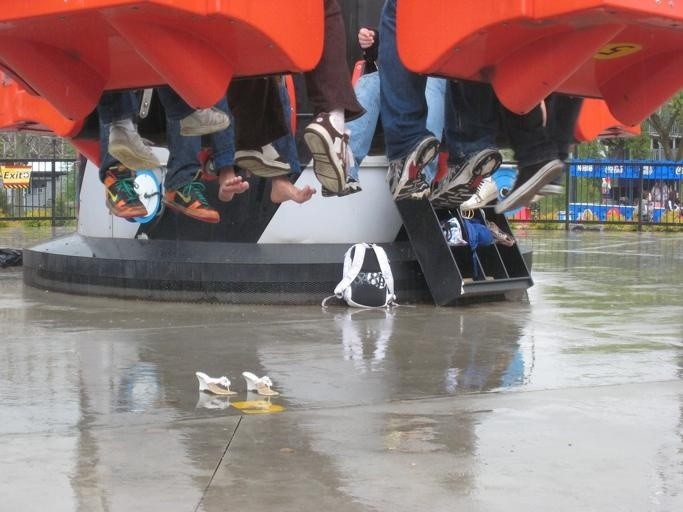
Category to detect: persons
[97,1,582,224]
[637,192,654,222]
[664,190,681,211]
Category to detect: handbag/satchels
[466,218,493,249]
[441,218,467,245]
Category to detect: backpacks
[335,243,396,309]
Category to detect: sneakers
[102,164,147,218]
[231,144,290,180]
[107,122,163,169]
[497,160,565,215]
[305,113,362,196]
[163,169,220,223]
[181,106,231,137]
[388,135,502,212]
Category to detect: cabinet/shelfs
[397,198,534,307]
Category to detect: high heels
[243,372,278,396]
[196,371,237,395]
